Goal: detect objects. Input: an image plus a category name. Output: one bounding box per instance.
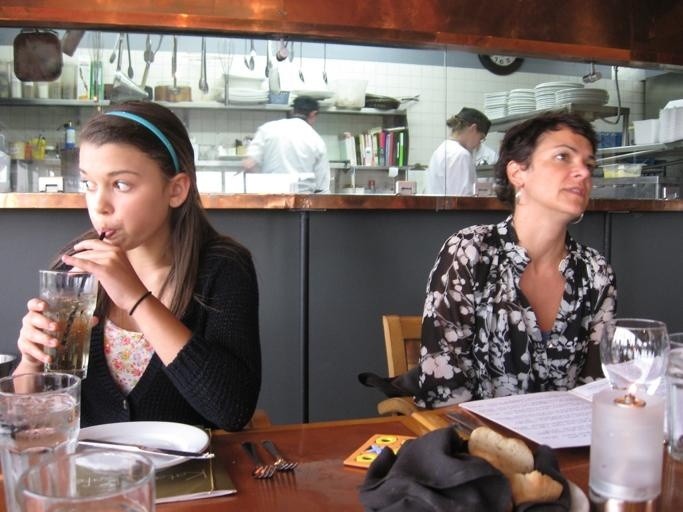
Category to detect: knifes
[78,439,215,460]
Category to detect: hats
[456,108,491,135]
[291,96,319,110]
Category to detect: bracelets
[129,291,151,317]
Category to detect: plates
[216,87,269,105]
[287,87,335,108]
[568,479,591,512]
[74,420,210,473]
[483,81,609,120]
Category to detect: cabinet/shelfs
[476,102,630,178]
[0,97,419,171]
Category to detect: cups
[589,388,664,503]
[665,348,683,462]
[598,317,669,396]
[0,371,83,512]
[17,451,156,512]
[38,267,99,378]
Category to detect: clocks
[478,53,524,75]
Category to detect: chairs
[376,314,423,417]
[212,410,272,434]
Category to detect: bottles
[64,120,76,149]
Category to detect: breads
[468,426,562,505]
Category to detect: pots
[365,92,420,110]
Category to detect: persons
[243,96,331,195]
[13,99,260,432]
[412,107,618,409]
[417,107,491,196]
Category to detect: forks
[241,440,298,479]
